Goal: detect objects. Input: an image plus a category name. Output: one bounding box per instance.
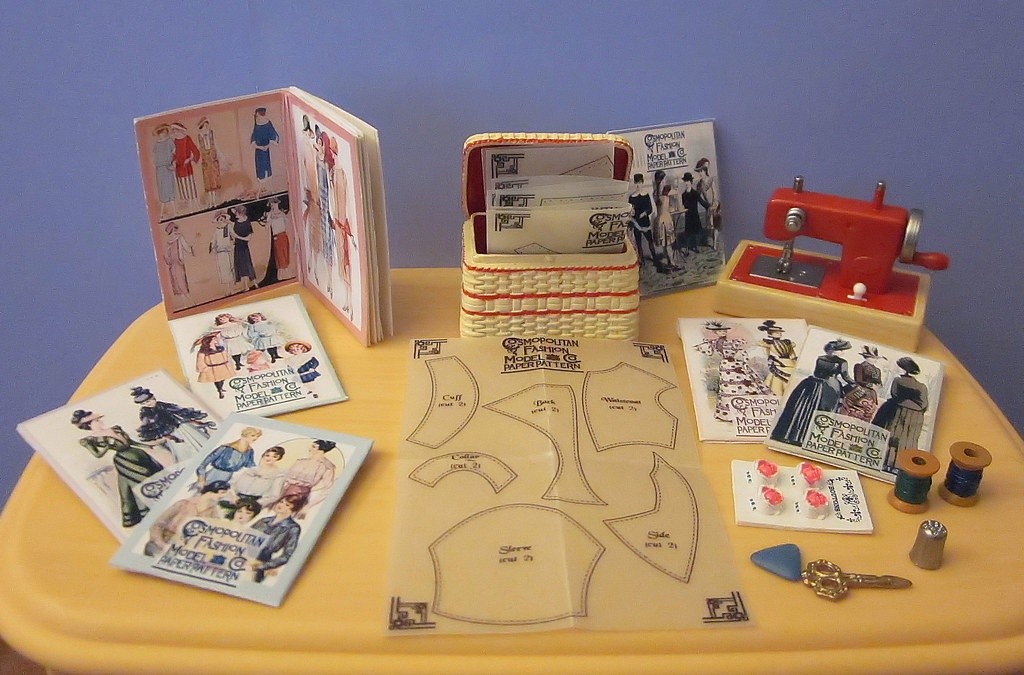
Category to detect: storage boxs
[455,130,645,343]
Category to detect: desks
[0,266,1024,675]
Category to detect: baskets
[460,132,640,340]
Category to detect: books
[131,86,394,347]
[607,118,725,297]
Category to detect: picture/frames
[607,118,725,300]
